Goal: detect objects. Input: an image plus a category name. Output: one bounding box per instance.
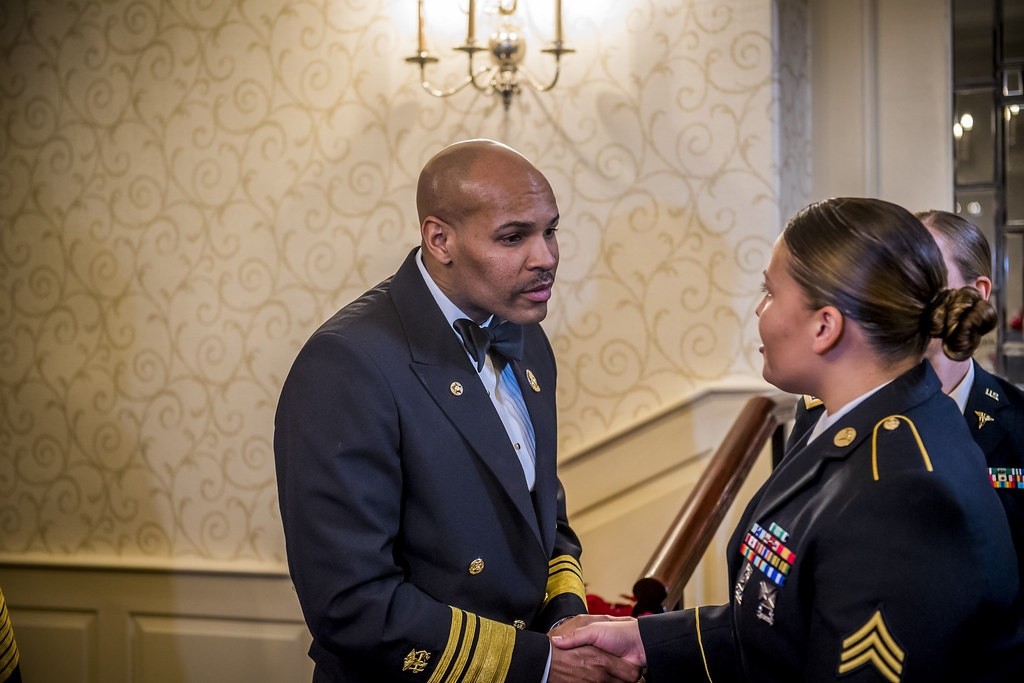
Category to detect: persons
[274,138,644,683]
[552,198,1023,682]
[914,212,1024,585]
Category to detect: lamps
[404,0,576,113]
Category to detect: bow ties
[452,318,525,373]
